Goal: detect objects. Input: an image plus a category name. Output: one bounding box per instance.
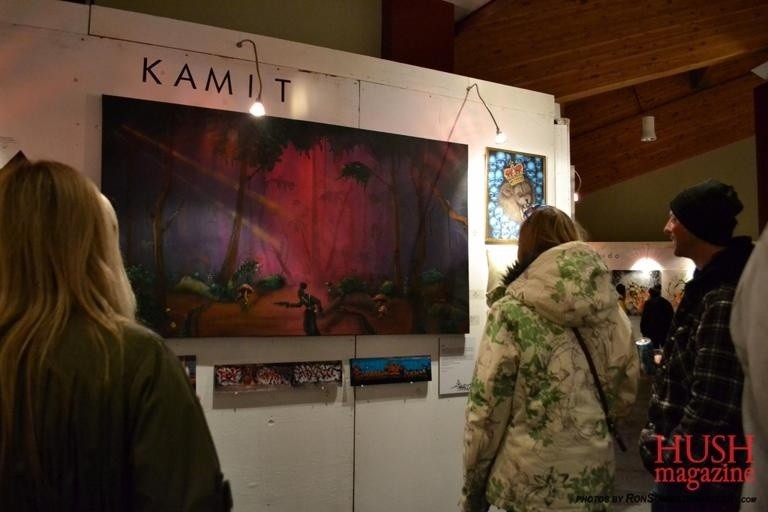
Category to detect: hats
[670,178,744,247]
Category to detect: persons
[635,177,759,512]
[0,157,235,512]
[454,202,641,511]
[271,282,326,337]
[639,281,674,375]
[727,222,767,511]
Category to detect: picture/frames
[483,146,548,248]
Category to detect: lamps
[640,114,656,143]
